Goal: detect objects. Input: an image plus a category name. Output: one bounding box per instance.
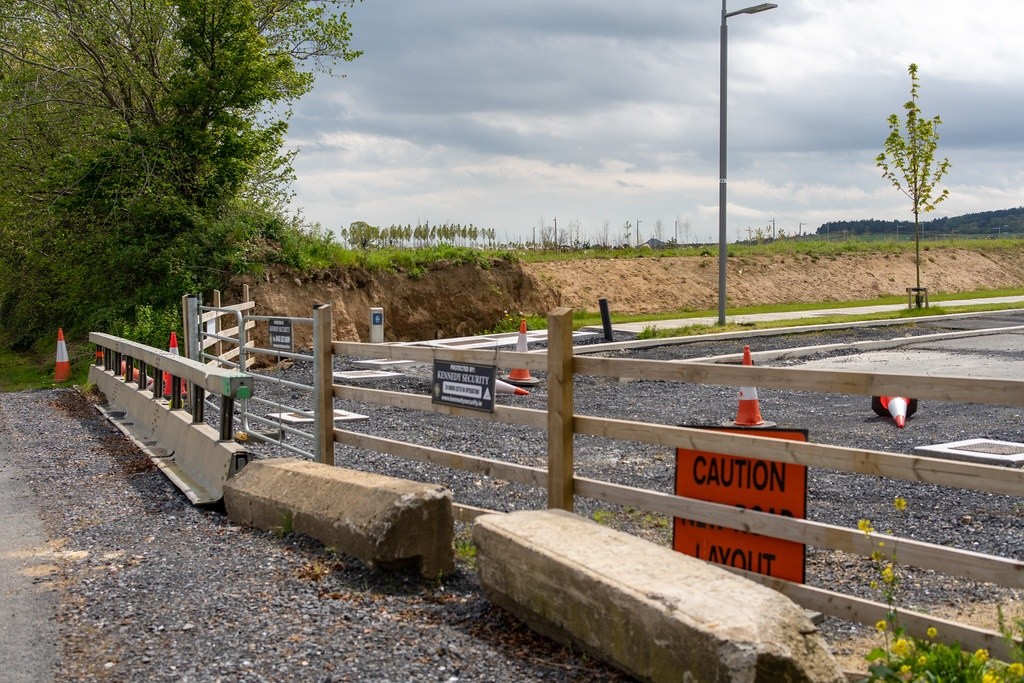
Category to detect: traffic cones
[871,396,920,429]
[495,378,529,396]
[54,328,72,381]
[733,346,766,426]
[507,319,531,381]
[121,360,153,385]
[161,331,187,399]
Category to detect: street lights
[717,1,778,327]
[529,212,933,254]
